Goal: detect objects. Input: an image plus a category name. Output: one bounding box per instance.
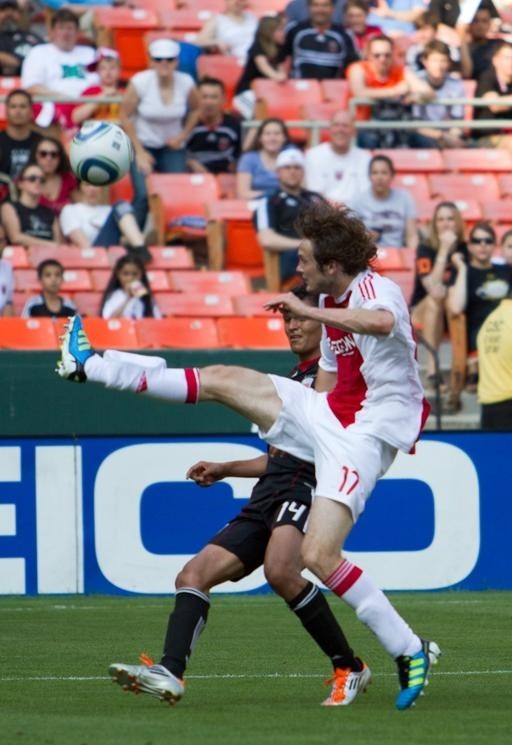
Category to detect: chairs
[0,0,512,348]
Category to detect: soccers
[69,118,134,187]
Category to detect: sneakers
[55,313,96,384]
[320,657,372,707]
[107,652,185,706]
[395,637,441,711]
[423,373,479,414]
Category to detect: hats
[276,148,304,171]
[84,51,120,72]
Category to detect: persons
[109,281,371,705]
[56,208,441,709]
[2,0,511,429]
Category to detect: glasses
[22,174,47,183]
[38,149,59,160]
[153,57,177,62]
[471,237,495,245]
